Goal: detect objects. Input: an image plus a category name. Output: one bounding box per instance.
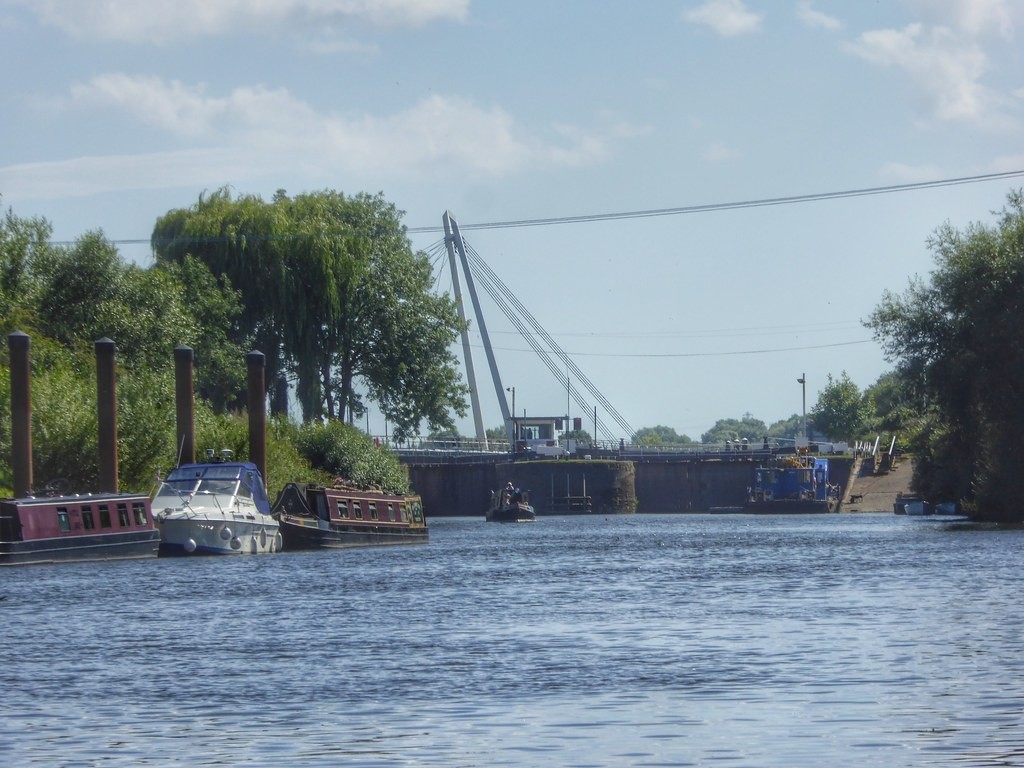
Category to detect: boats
[742,455,843,514]
[485,489,536,525]
[149,448,284,556]
[278,481,429,550]
[0,485,162,569]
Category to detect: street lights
[506,386,515,463]
[796,372,807,441]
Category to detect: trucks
[536,445,570,460]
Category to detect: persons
[504,482,522,504]
